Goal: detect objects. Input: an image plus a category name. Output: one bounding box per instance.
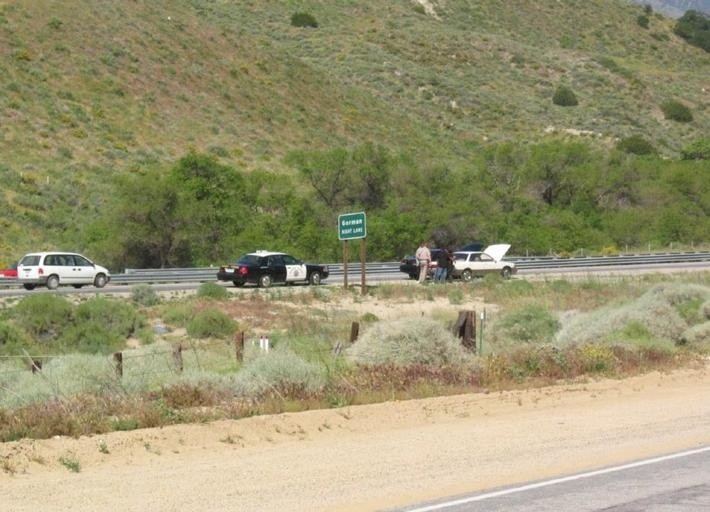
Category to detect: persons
[447,250,454,281]
[433,248,456,282]
[415,240,431,284]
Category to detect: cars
[400,243,517,282]
[217,251,330,288]
[17,252,111,290]
